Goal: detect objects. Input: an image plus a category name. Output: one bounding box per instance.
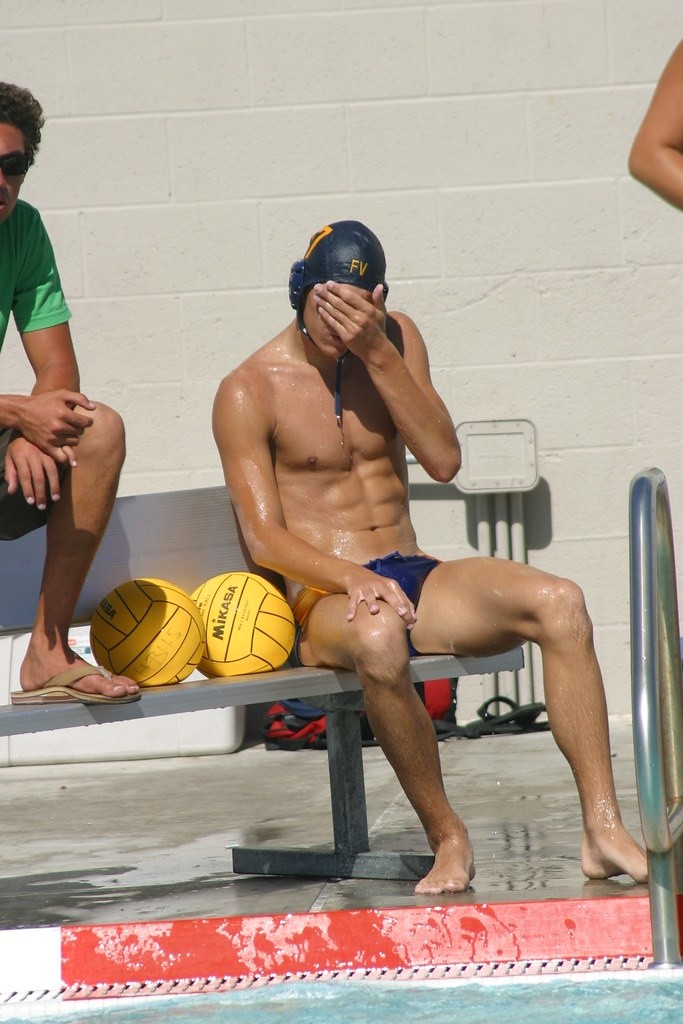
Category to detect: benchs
[0,485,522,894]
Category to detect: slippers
[10,665,142,705]
[465,696,550,739]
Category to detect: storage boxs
[0,625,246,766]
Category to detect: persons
[0,80,141,702]
[212,221,648,892]
[628,39,683,212]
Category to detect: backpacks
[259,675,459,749]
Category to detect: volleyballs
[188,571,295,678]
[90,579,206,686]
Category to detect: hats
[290,219,390,309]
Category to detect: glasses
[0,155,33,177]
